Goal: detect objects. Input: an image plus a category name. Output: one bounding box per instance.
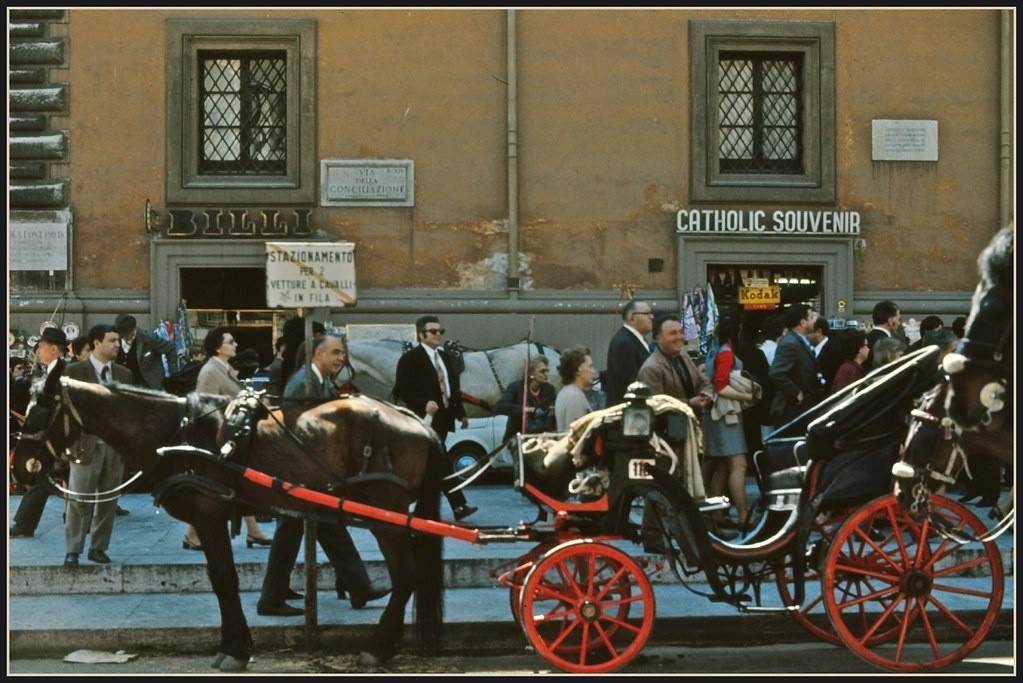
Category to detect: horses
[11,359,449,674]
[328,338,565,421]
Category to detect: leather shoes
[257,599,303,617]
[88,548,110,564]
[349,585,393,609]
[64,553,80,567]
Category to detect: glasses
[532,367,550,374]
[421,328,444,335]
[223,339,236,346]
[631,311,654,316]
[14,367,25,371]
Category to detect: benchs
[754,381,900,512]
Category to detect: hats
[113,314,136,339]
[41,327,72,345]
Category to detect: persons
[554,299,712,601]
[695,300,1014,542]
[181,329,272,549]
[498,354,557,475]
[256,335,391,616]
[10,314,178,566]
[396,315,478,520]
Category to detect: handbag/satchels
[526,403,557,432]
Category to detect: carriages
[12,344,1003,670]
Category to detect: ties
[435,351,449,409]
[102,366,108,382]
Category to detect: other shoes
[958,485,999,507]
[117,506,129,516]
[454,505,479,521]
[856,530,885,542]
[644,543,680,555]
[714,515,761,541]
[9,521,34,537]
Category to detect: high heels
[335,580,349,600]
[284,589,304,599]
[245,534,272,549]
[181,535,203,550]
[988,505,1013,533]
